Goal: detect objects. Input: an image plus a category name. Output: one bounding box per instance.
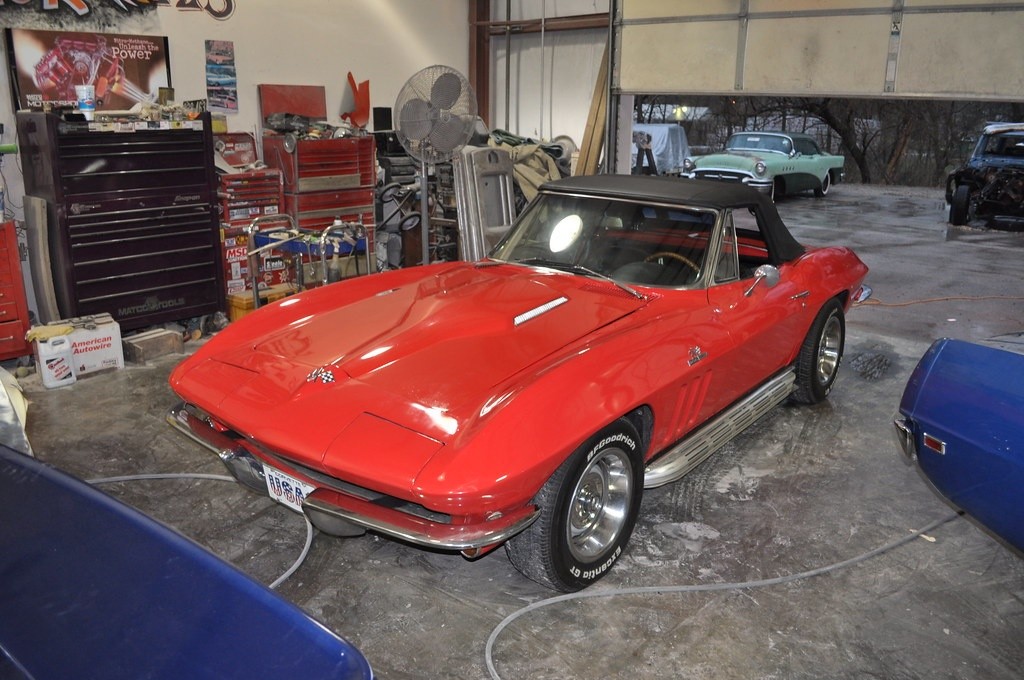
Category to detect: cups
[75,84,95,121]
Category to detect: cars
[944,122,1024,230]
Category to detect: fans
[391,65,479,267]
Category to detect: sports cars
[164,173,873,595]
[679,131,845,206]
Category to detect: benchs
[588,237,771,287]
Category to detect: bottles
[332,217,343,233]
[36,334,77,388]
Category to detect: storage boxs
[227,283,302,324]
[48,310,125,378]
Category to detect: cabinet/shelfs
[0,221,33,364]
[20,108,228,333]
[262,134,376,287]
